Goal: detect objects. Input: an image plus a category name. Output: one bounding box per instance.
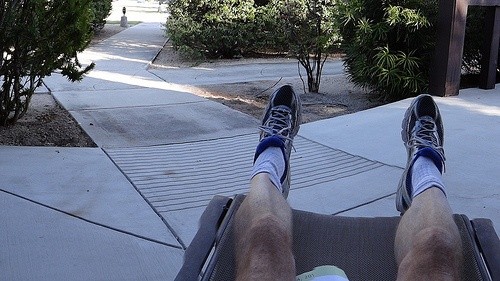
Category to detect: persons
[218,82,464,281]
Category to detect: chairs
[164,192,500,281]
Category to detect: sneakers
[253,85,302,201]
[395,94,445,213]
